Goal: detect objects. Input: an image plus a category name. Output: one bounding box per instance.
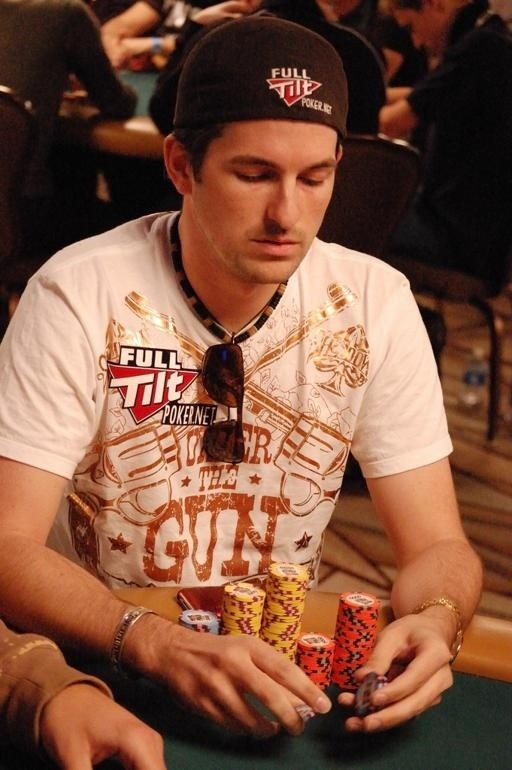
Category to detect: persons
[0,617,169,769]
[0,15,489,746]
[0,1,512,287]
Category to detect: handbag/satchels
[410,596,465,668]
[111,606,157,681]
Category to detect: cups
[202,342,245,465]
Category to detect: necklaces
[169,211,288,345]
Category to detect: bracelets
[410,596,465,668]
[111,606,157,681]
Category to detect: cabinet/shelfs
[456,347,490,422]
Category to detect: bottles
[175,8,349,136]
